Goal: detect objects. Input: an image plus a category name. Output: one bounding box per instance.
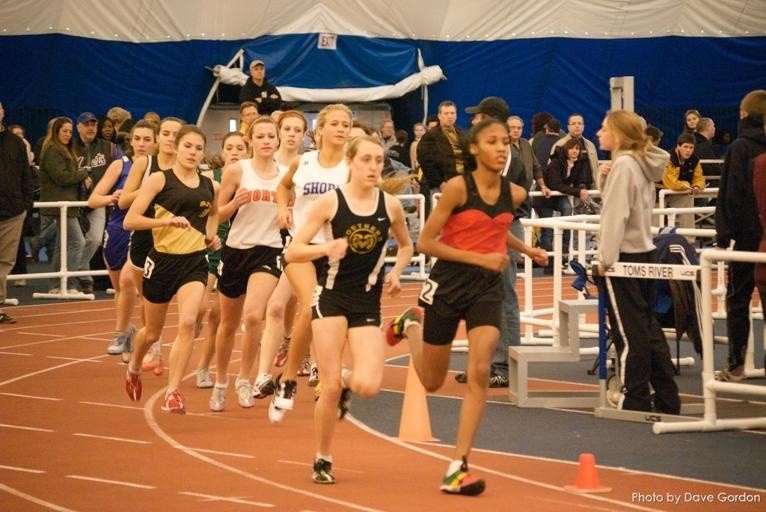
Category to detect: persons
[369,116,440,179]
[251,110,308,399]
[683,109,701,133]
[273,292,313,376]
[645,126,664,146]
[532,137,590,275]
[267,103,353,424]
[547,113,602,213]
[196,131,249,388]
[98,105,160,156]
[533,113,567,156]
[533,119,567,167]
[29,137,57,262]
[505,115,551,218]
[122,125,221,415]
[117,117,187,376]
[694,117,719,187]
[0,101,35,324]
[455,96,521,388]
[416,100,471,264]
[208,117,290,412]
[8,124,36,287]
[284,136,415,484]
[715,90,766,383]
[39,116,91,293]
[713,128,732,157]
[385,116,549,495]
[239,102,260,143]
[309,121,368,401]
[87,120,157,363]
[72,111,111,294]
[654,132,706,203]
[596,110,681,415]
[238,59,281,115]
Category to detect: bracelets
[542,185,547,188]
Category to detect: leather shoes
[28,239,39,262]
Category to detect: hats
[249,60,265,68]
[464,96,510,121]
[78,112,96,123]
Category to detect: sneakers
[107,332,126,354]
[141,337,161,371]
[0,313,15,323]
[715,368,747,382]
[272,373,296,411]
[125,365,142,401]
[338,388,353,420]
[160,387,186,415]
[312,459,336,483]
[252,372,273,399]
[308,366,320,387]
[196,367,213,387]
[121,351,130,363]
[607,388,624,409]
[78,277,93,293]
[234,376,258,408]
[440,455,485,494]
[268,401,285,423]
[273,337,290,367]
[297,357,311,376]
[209,374,228,411]
[455,372,467,383]
[489,373,508,387]
[385,306,423,346]
